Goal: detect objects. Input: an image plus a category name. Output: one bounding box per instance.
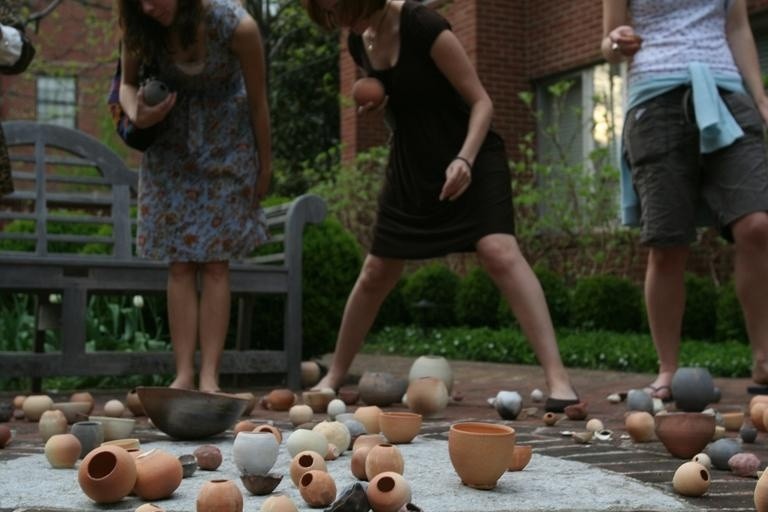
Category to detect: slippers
[645,385,671,401]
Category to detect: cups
[378,412,423,444]
[73,422,102,458]
[448,423,517,490]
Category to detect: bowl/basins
[654,414,714,457]
[88,416,137,443]
[137,386,250,441]
[55,400,93,422]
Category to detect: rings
[611,42,622,52]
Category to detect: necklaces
[366,1,392,51]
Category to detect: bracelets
[455,156,473,169]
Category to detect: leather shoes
[546,386,579,413]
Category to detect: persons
[598,1,768,399]
[297,1,580,412]
[0,20,36,195]
[118,1,272,394]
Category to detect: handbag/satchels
[107,57,171,152]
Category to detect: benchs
[1,121,328,400]
